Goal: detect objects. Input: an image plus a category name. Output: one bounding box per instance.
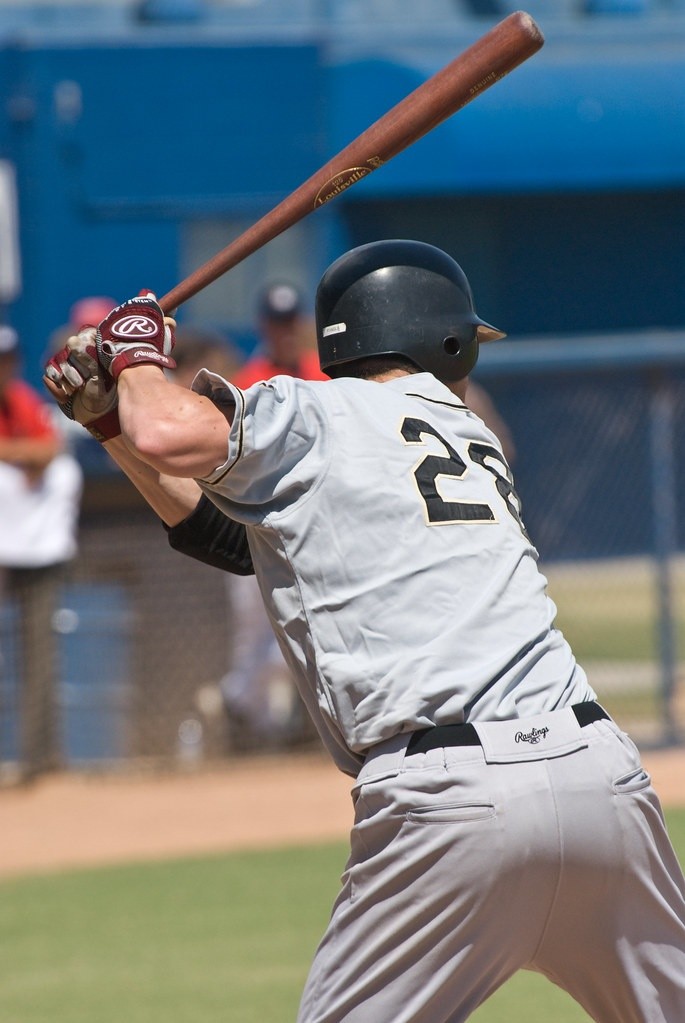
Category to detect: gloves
[44,288,177,445]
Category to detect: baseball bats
[41,9,545,404]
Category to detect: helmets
[315,240,506,386]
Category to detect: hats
[255,279,304,322]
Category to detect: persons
[0,281,335,788]
[42,241,685,1023]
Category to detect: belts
[402,703,612,757]
